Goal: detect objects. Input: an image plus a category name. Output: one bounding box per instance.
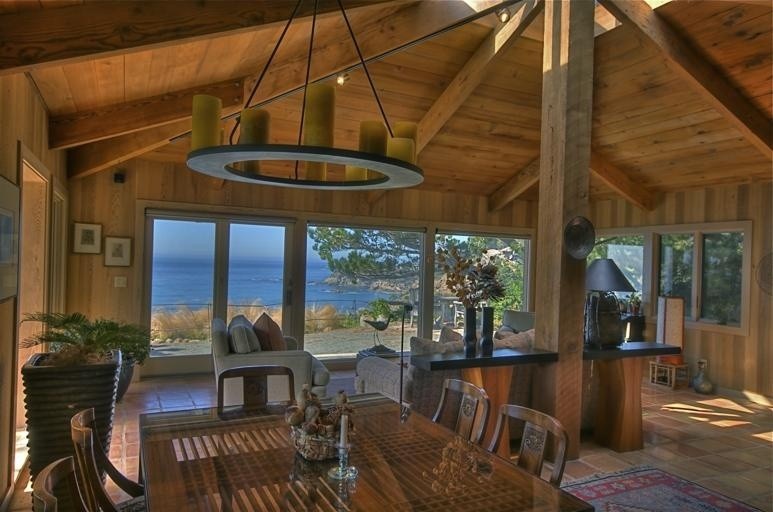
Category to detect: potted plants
[18,305,151,403]
[625,292,642,315]
[617,298,627,311]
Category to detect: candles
[337,412,349,448]
[186,80,422,184]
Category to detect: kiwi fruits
[284,387,354,437]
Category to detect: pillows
[409,334,443,359]
[227,314,261,353]
[501,308,536,337]
[438,323,463,346]
[253,311,287,353]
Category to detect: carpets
[558,462,769,512]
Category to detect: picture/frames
[71,220,103,254]
[101,235,134,268]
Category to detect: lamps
[583,257,635,349]
[653,295,685,368]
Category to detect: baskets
[292,431,351,460]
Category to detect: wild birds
[364,314,395,346]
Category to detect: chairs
[69,406,148,511]
[427,377,491,447]
[488,402,570,487]
[208,312,330,410]
[406,286,487,332]
[29,451,92,511]
[214,366,296,510]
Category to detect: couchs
[354,311,535,409]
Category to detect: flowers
[457,264,505,306]
[436,244,506,307]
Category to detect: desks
[581,338,681,454]
[135,391,595,511]
[406,348,557,461]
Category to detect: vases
[479,306,497,356]
[691,360,714,395]
[459,306,478,357]
[20,346,123,494]
